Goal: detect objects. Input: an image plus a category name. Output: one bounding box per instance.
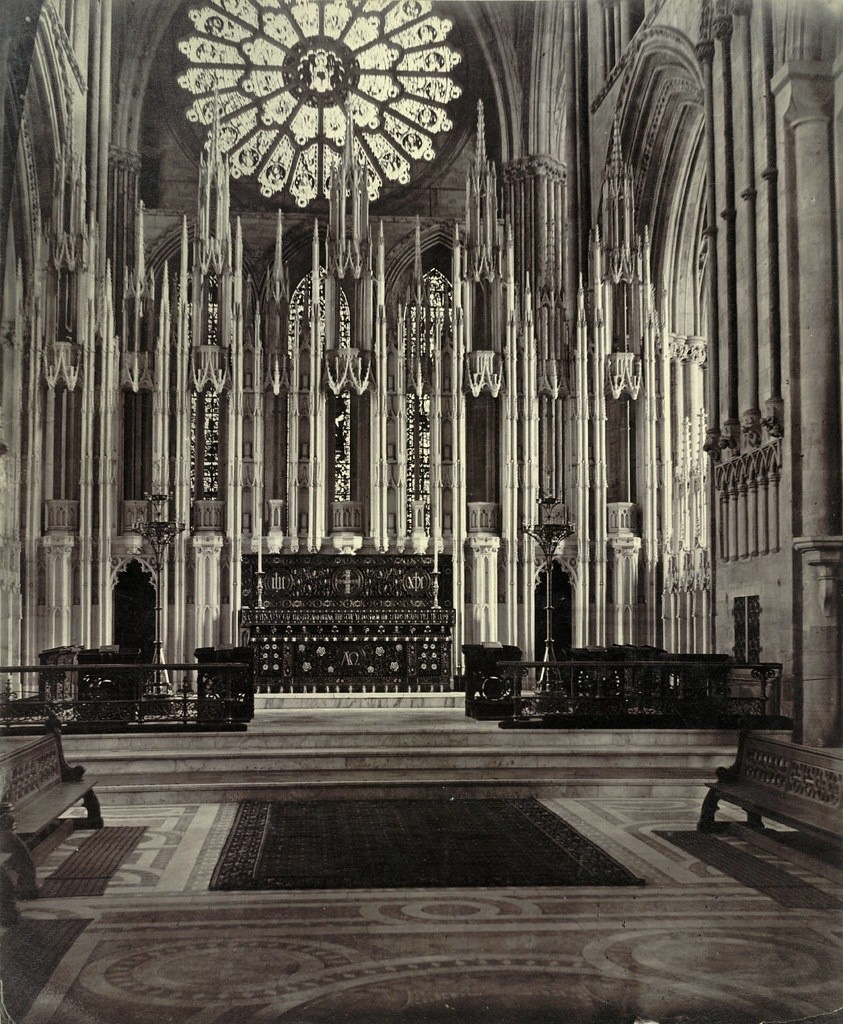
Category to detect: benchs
[695,729,843,851]
[0,725,104,893]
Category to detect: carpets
[206,798,648,893]
[0,918,95,1024]
[651,830,843,909]
[38,826,149,899]
